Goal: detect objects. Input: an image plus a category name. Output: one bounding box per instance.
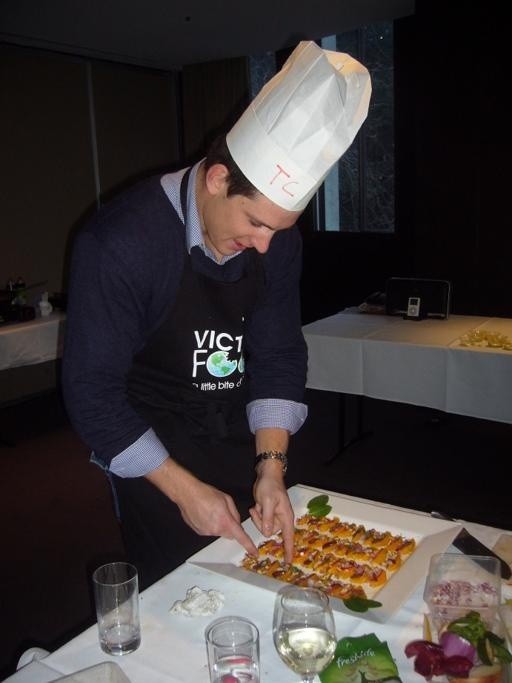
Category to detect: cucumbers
[477,638,494,667]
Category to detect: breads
[447,656,503,683]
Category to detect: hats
[225,39,372,211]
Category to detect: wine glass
[272,585,338,683]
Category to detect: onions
[405,631,475,681]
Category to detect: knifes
[430,509,512,581]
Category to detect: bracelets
[254,451,288,473]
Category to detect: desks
[301,305,512,467]
[0,483,512,683]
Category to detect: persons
[53,134,312,599]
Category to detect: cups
[92,561,142,657]
[204,615,260,683]
[460,328,512,350]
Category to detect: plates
[184,487,461,627]
